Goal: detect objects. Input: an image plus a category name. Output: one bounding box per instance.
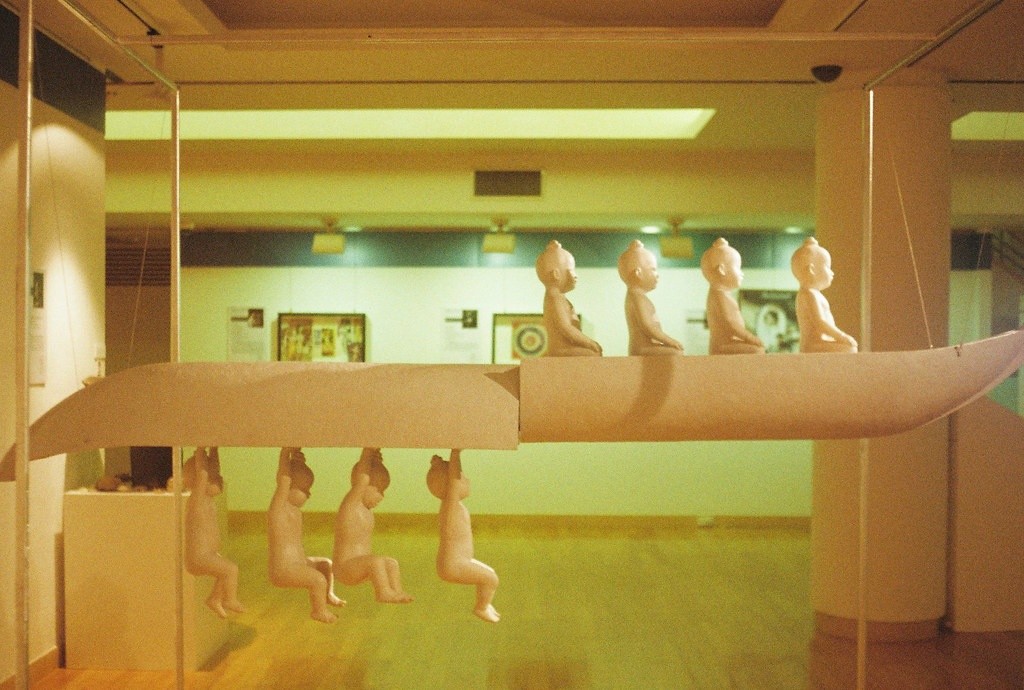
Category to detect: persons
[618,239,685,356]
[758,306,786,354]
[332,445,414,604]
[700,237,765,354]
[283,324,361,362]
[427,449,500,622]
[267,446,347,623]
[249,311,258,327]
[536,240,603,356]
[183,446,246,619]
[791,236,859,352]
[115,447,173,490]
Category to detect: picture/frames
[492,313,580,365]
[277,313,366,363]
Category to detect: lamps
[482,217,519,255]
[310,217,345,255]
[661,213,695,260]
